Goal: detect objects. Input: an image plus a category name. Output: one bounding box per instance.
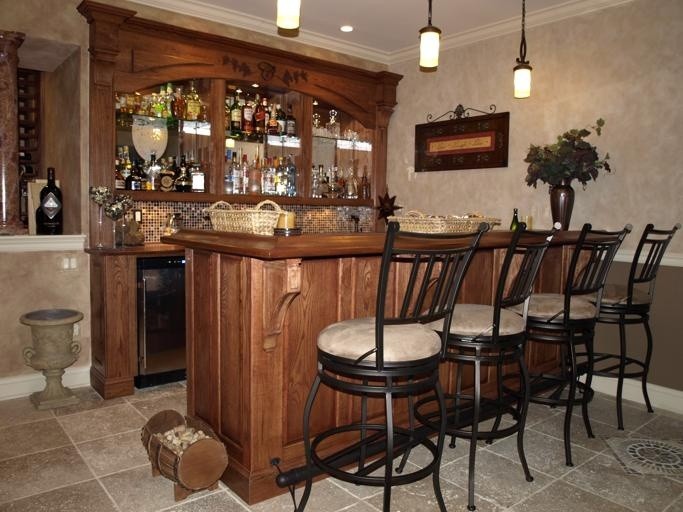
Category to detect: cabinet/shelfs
[78,0,405,203]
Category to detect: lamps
[513,0,532,99]
[418,0,441,68]
[275,0,301,30]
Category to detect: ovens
[134,259,186,389]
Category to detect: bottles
[311,159,370,200]
[165,212,181,237]
[116,145,205,193]
[510,207,520,230]
[225,146,298,197]
[38,166,63,234]
[312,109,341,137]
[225,86,297,138]
[116,80,209,123]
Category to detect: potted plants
[524,118,611,230]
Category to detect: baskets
[387,216,502,233]
[204,200,287,236]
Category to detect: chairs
[421,217,561,512]
[504,223,634,465]
[280,220,490,512]
[583,222,682,431]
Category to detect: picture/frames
[415,105,509,172]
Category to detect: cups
[277,212,296,230]
[521,215,533,231]
[343,129,359,141]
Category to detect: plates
[131,125,168,161]
